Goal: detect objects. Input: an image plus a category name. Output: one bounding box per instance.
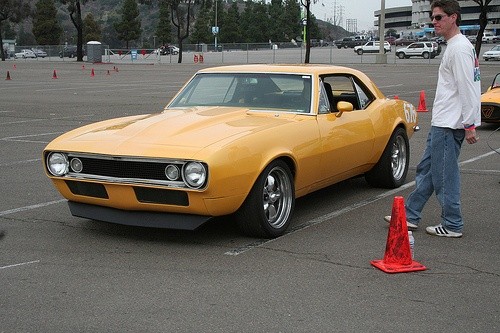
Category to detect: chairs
[237,83,264,103]
[299,81,335,107]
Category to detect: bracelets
[464,123,476,132]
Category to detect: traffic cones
[90,68,96,76]
[369,195,427,274]
[52,69,58,79]
[4,71,12,80]
[81,64,86,70]
[416,90,429,112]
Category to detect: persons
[382,0,482,237]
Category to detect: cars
[59,45,85,58]
[482,44,500,61]
[309,39,329,47]
[334,33,500,51]
[153,45,180,55]
[13,46,48,59]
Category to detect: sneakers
[426,223,462,238]
[384,215,418,230]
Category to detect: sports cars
[41,63,421,239]
[480,72,500,123]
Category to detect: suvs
[354,40,391,55]
[395,40,442,59]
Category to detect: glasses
[430,13,450,21]
